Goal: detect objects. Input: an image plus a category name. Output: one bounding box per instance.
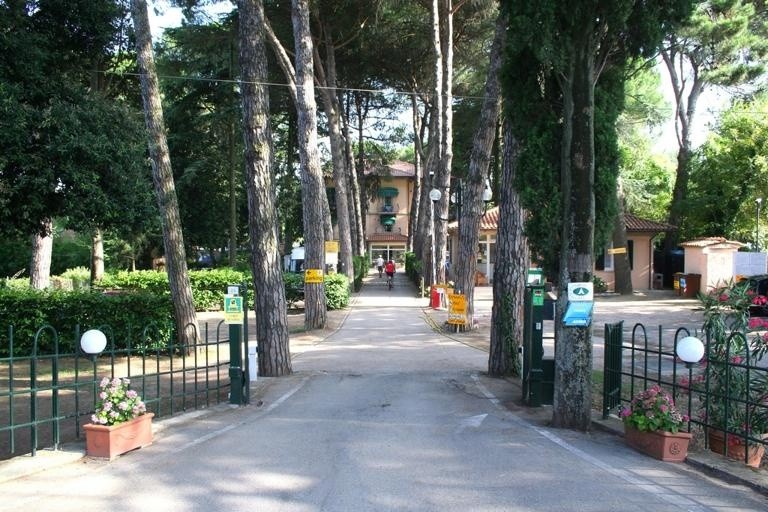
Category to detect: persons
[384,260,396,288]
[375,254,385,278]
[391,256,396,269]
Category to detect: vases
[623,424,693,463]
[82,412,155,459]
[707,426,765,467]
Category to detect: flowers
[620,385,690,434]
[673,275,768,446]
[90,375,147,426]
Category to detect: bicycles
[387,276,393,290]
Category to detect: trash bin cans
[653,273,665,289]
[432,285,448,311]
[672,272,701,299]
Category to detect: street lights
[429,187,492,248]
